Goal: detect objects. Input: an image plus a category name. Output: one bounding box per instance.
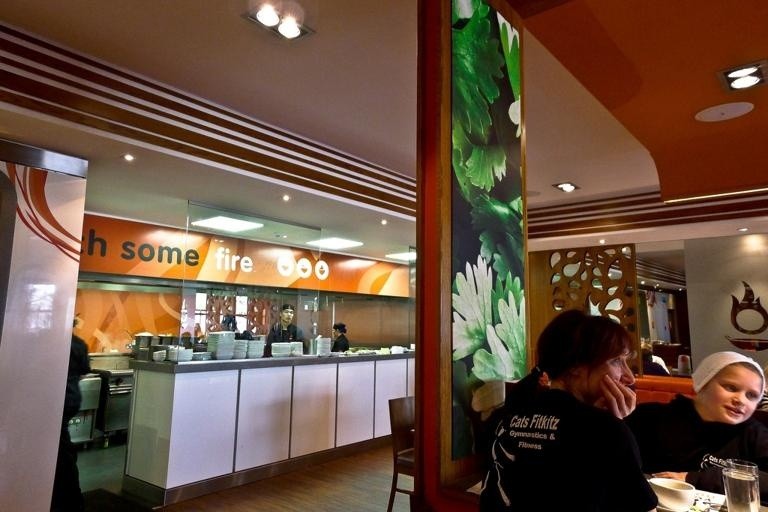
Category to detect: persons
[478,308,658,511]
[641,343,671,376]
[266,304,309,356]
[59,314,92,472]
[330,322,350,351]
[637,349,767,471]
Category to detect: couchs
[635,377,696,410]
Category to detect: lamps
[250,0,312,43]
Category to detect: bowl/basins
[648,477,696,511]
[152,350,166,361]
[316,337,331,356]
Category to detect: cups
[721,468,760,512]
[726,458,758,475]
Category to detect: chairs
[384,395,418,510]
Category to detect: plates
[192,351,212,360]
[270,342,291,357]
[168,349,193,361]
[247,340,264,357]
[208,330,235,359]
[234,340,248,358]
[290,341,303,356]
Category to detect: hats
[279,304,294,311]
[692,350,765,404]
[333,322,346,328]
